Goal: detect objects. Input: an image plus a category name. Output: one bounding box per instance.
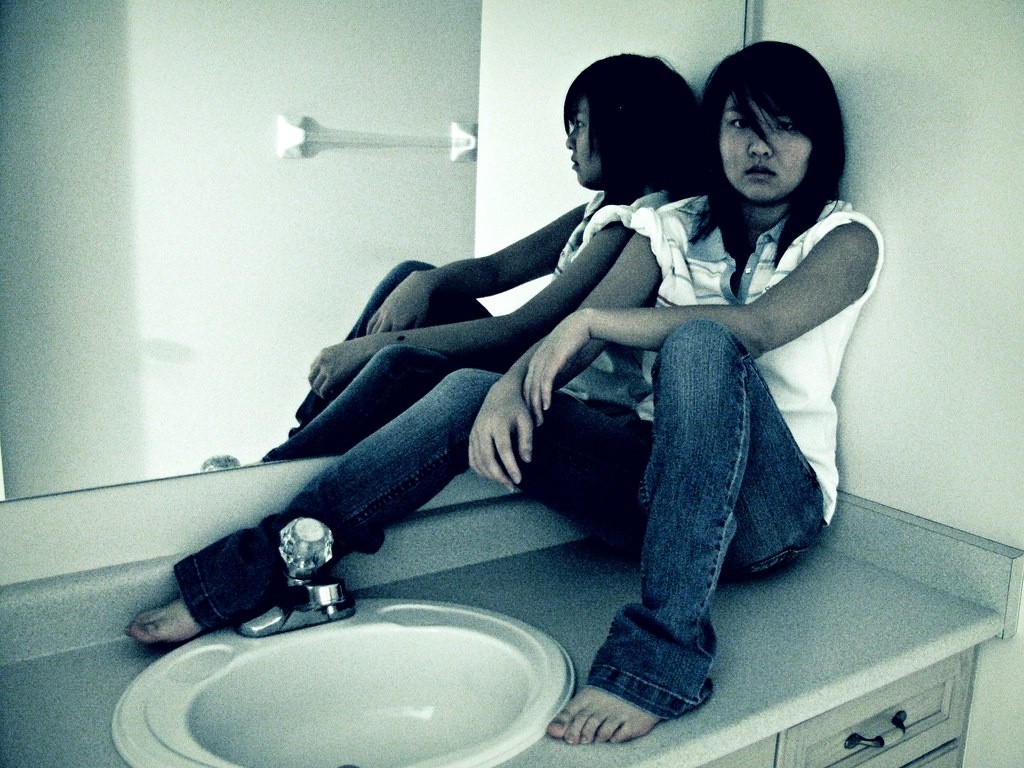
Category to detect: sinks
[111,598,575,768]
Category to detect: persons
[256,53,699,462]
[126,39,884,745]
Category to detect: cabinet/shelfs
[767,642,995,768]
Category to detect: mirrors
[0,0,763,503]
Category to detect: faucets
[243,517,356,637]
[202,454,239,473]
